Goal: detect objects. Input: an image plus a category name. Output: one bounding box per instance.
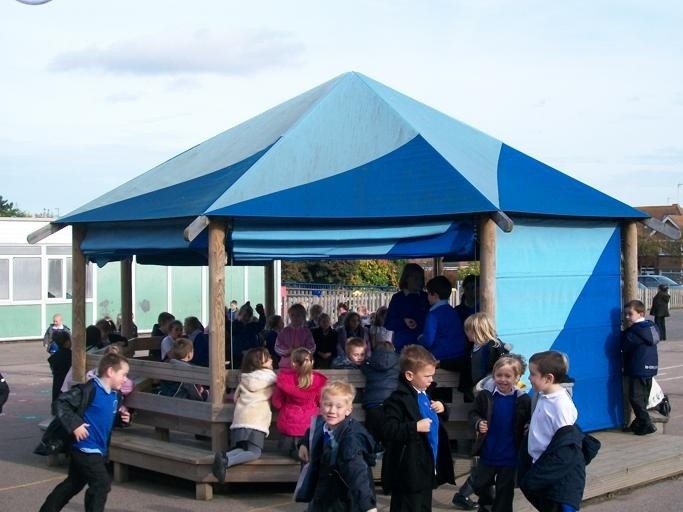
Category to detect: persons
[451,312,601,512]
[292,379,373,512]
[213,261,478,482]
[651,284,670,341]
[374,345,449,512]
[38,352,131,511]
[620,300,660,435]
[149,313,209,401]
[1,374,10,416]
[42,313,138,415]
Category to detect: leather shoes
[623,421,636,432]
[452,494,481,510]
[634,424,657,435]
[211,450,227,483]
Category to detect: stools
[636,406,668,432]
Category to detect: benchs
[217,369,382,483]
[37,354,218,500]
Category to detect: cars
[636,275,683,288]
